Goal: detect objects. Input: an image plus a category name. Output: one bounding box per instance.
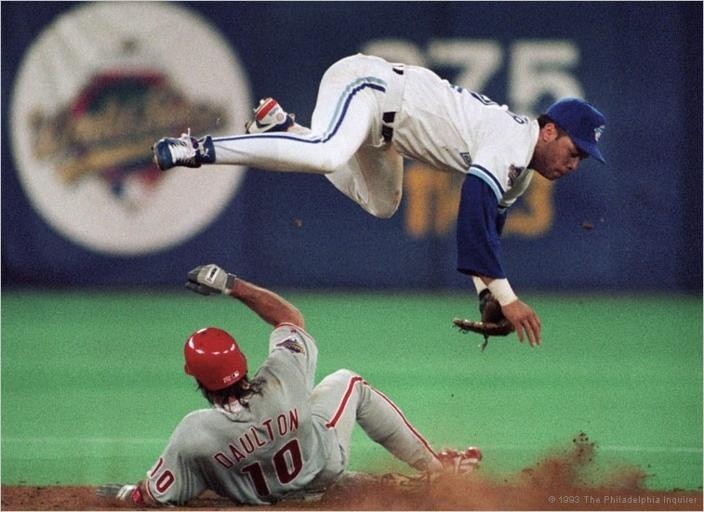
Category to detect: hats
[546,96,608,166]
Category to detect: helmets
[184,326,247,391]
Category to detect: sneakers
[245,96,294,134]
[152,133,216,171]
[427,446,481,487]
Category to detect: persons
[149,50,609,351]
[94,261,484,507]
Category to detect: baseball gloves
[454,292,514,336]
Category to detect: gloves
[95,483,140,504]
[185,263,238,297]
